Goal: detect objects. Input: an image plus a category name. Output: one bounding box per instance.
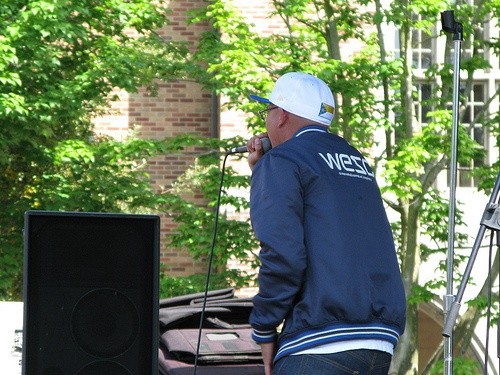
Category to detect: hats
[249,73,335,125]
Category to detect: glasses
[258,106,279,121]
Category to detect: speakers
[21,209,161,375]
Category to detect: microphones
[228,137,272,156]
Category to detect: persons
[247,72,407,375]
